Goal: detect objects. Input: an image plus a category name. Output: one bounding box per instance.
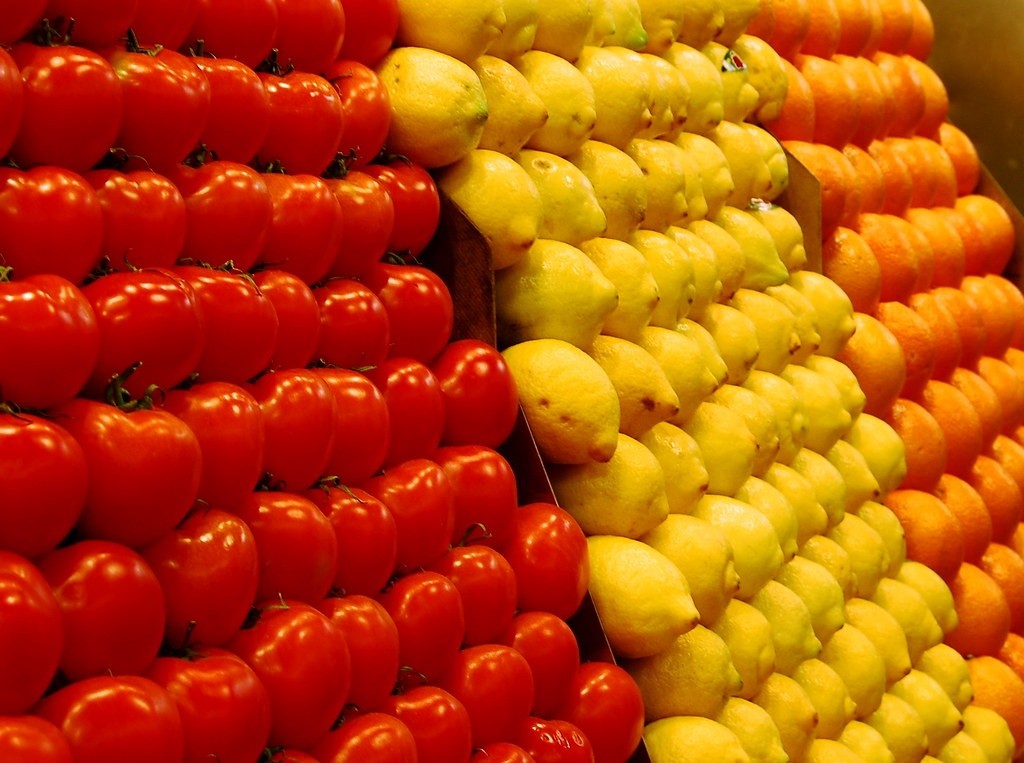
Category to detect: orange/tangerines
[748,0,1024,763]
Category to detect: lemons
[380,0,1013,763]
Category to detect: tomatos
[0,0,644,763]
